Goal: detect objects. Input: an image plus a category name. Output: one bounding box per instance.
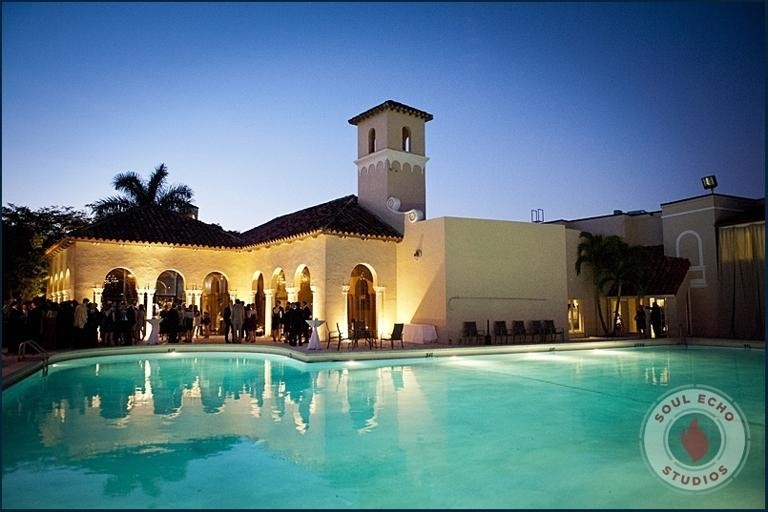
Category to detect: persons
[633,302,664,338]
[1,297,312,347]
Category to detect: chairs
[324,317,408,353]
[459,315,568,346]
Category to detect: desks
[401,323,437,345]
